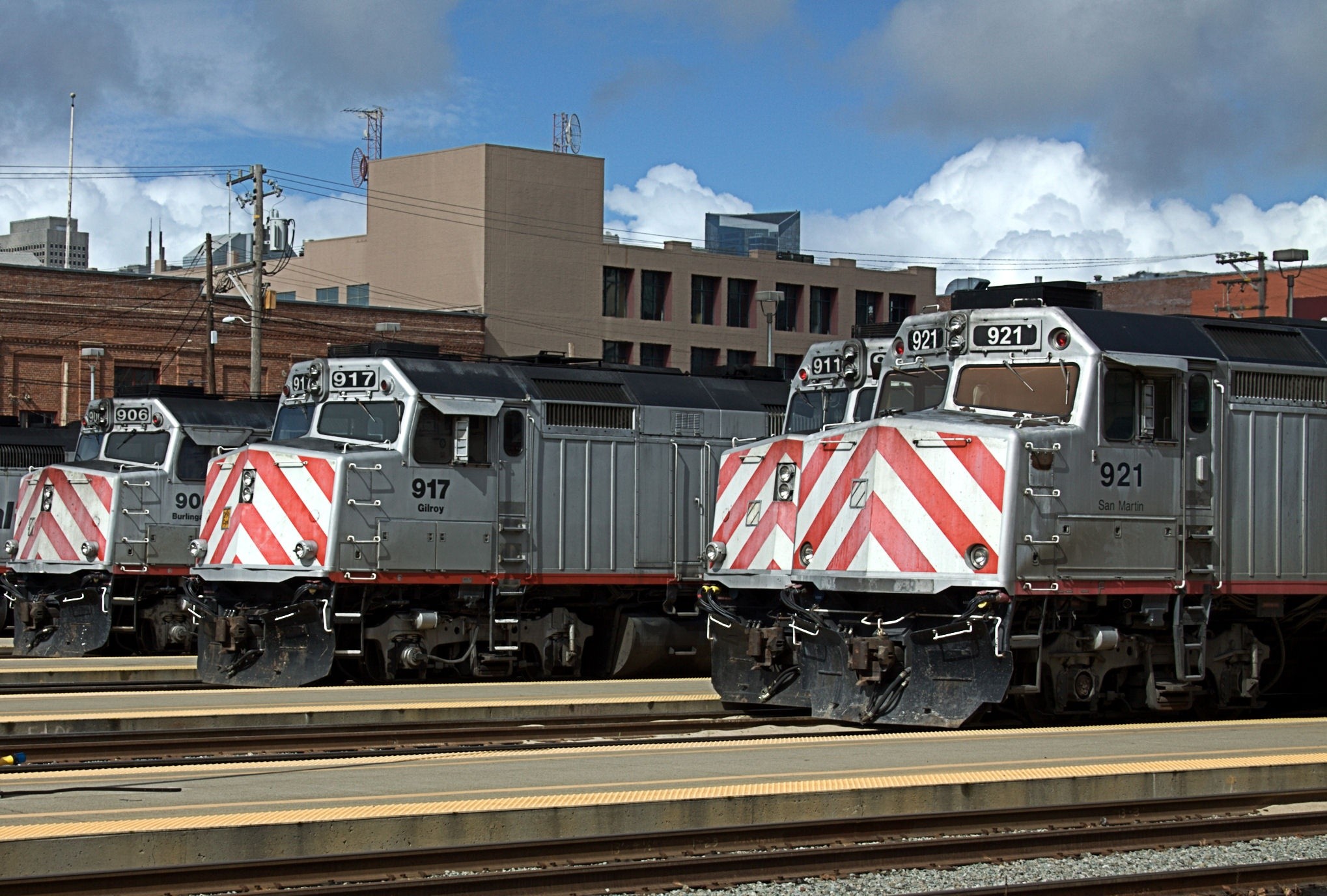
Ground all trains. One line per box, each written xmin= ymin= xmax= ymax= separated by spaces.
xmin=698 ymin=277 xmax=1325 ymax=728
xmin=1 ymin=348 xmax=815 ymax=686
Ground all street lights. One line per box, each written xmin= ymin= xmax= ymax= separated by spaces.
xmin=1273 ymin=249 xmax=1309 ymax=317
xmin=221 ymin=316 xmax=263 ymax=399
xmin=755 ymin=290 xmax=785 ymax=367
xmin=80 ymin=346 xmax=104 ymax=401
